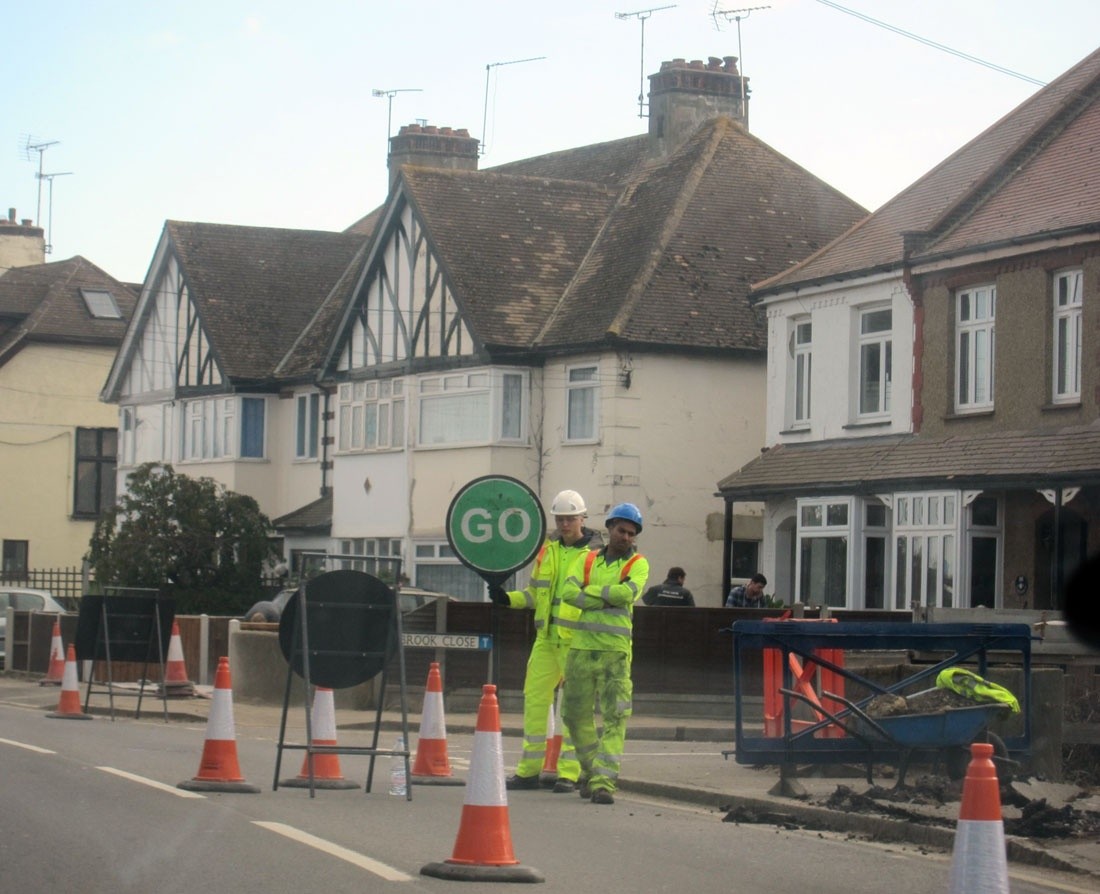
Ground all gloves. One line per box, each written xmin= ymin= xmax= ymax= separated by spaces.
xmin=488 ymin=585 xmax=510 ymax=606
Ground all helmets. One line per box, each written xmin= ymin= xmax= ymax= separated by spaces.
xmin=605 ymin=503 xmax=642 ymax=534
xmin=550 ymin=490 xmax=587 ymax=516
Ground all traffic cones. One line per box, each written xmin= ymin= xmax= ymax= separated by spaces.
xmin=419 ymin=682 xmax=546 ymax=881
xmin=280 ymin=684 xmax=362 ymax=790
xmin=158 ymin=619 xmax=195 ymax=698
xmin=536 ymin=680 xmax=567 ymax=782
xmin=944 ymin=743 xmax=1011 ymax=894
xmin=408 ymin=661 xmax=466 ymax=785
xmin=45 ymin=643 xmax=94 ymax=721
xmin=178 ymin=656 xmax=261 ymax=794
xmin=40 ymin=620 xmax=69 ymax=684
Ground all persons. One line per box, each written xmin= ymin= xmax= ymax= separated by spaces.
xmin=487 ymin=489 xmax=606 ymax=793
xmin=642 ymin=567 xmax=695 ymax=607
xmin=725 ymin=574 xmax=769 ymax=609
xmin=560 ymin=503 xmax=649 ymax=804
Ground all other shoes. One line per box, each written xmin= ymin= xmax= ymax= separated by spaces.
xmin=580 ymin=773 xmax=592 ymax=798
xmin=506 ymin=775 xmax=540 ymax=789
xmin=553 ymin=777 xmax=575 ymax=793
xmin=592 ymin=787 xmax=615 ymax=804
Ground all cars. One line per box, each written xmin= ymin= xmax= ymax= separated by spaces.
xmin=0 ymin=586 xmax=69 ymax=632
